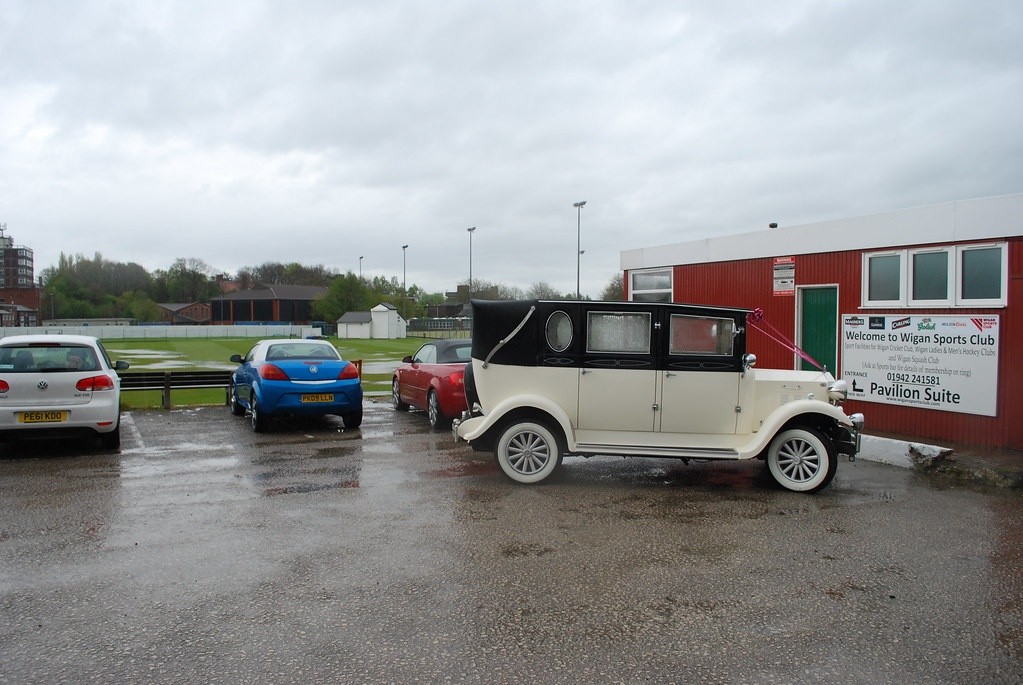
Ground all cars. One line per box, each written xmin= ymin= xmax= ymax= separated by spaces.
xmin=391 ymin=338 xmax=475 ymax=431
xmin=0 ymin=334 xmax=130 ymax=451
xmin=228 ymin=338 xmax=364 ymax=434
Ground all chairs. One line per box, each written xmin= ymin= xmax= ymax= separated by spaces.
xmin=309 ymin=349 xmax=327 ymax=356
xmin=16 ymin=351 xmax=34 ymax=368
xmin=67 ymin=349 xmax=89 ymax=367
xmin=271 ymin=349 xmax=292 ymax=357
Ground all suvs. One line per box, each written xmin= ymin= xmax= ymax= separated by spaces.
xmin=448 ymin=298 xmax=865 ymax=493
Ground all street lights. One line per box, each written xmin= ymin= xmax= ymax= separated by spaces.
xmin=467 ymin=226 xmax=476 ymax=298
xmin=359 ymin=257 xmax=363 ymax=309
xmin=572 ymin=199 xmax=587 ymax=297
xmin=402 ymin=244 xmax=409 ymax=317
